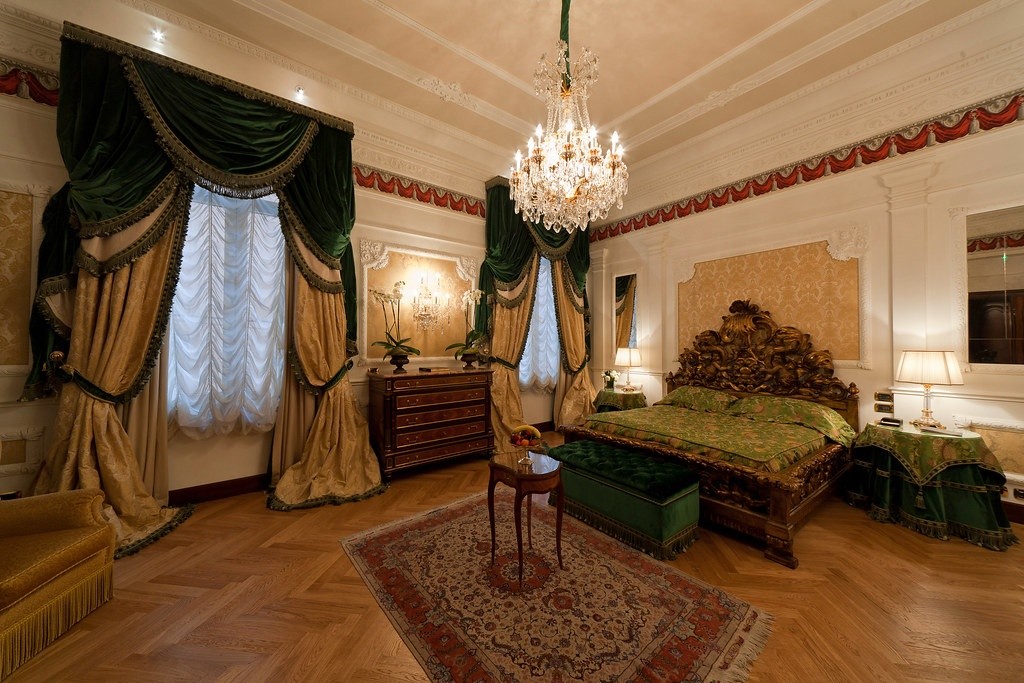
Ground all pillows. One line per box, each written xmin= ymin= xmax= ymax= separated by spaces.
xmin=727 ymin=396 xmax=855 ymax=447
xmin=653 ymin=385 xmax=739 ymax=414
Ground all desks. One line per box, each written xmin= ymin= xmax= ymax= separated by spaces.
xmin=593 ymin=386 xmax=646 ymax=412
xmin=849 ymin=421 xmax=985 ymax=551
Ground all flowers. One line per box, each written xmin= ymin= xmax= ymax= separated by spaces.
xmin=445 ymin=289 xmax=492 ymax=360
xmin=601 ymin=370 xmax=620 ymax=384
xmin=368 ymin=282 xmax=420 ymax=361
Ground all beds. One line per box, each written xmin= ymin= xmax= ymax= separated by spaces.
xmin=558 ymin=299 xmax=859 ymax=570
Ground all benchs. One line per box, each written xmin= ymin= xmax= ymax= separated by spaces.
xmin=547 ymin=439 xmax=699 ymax=560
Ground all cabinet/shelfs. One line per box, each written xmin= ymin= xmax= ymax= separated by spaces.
xmin=368 ymin=368 xmax=497 ymax=488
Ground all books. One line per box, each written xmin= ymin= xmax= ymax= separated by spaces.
xmin=920 ymin=426 xmax=962 ymax=437
xmin=419 ymin=367 xmax=449 ymax=372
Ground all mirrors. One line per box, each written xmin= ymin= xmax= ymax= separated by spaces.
xmin=948 ymin=196 xmax=1024 ymax=374
xmin=611 ymin=267 xmax=641 ymax=360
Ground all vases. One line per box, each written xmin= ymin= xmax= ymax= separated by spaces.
xmin=461 ymin=354 xmax=479 ymax=370
xmin=391 ymin=355 xmax=409 ymax=373
xmin=605 ymin=381 xmax=614 ymax=391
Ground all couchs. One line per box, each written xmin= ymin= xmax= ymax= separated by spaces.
xmin=0 ymin=488 xmax=114 ymax=682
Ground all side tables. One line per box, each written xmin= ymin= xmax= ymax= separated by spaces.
xmin=488 ymin=450 xmax=564 ymax=583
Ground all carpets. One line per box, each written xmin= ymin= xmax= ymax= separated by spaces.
xmin=340 ymin=488 xmax=776 ymax=683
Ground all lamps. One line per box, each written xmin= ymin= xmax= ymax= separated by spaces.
xmin=509 ymin=0 xmax=629 ymax=234
xmin=411 ymin=266 xmax=451 ymax=336
xmin=895 ymin=351 xmax=963 ymax=429
xmin=614 ymin=348 xmax=641 ymax=391
